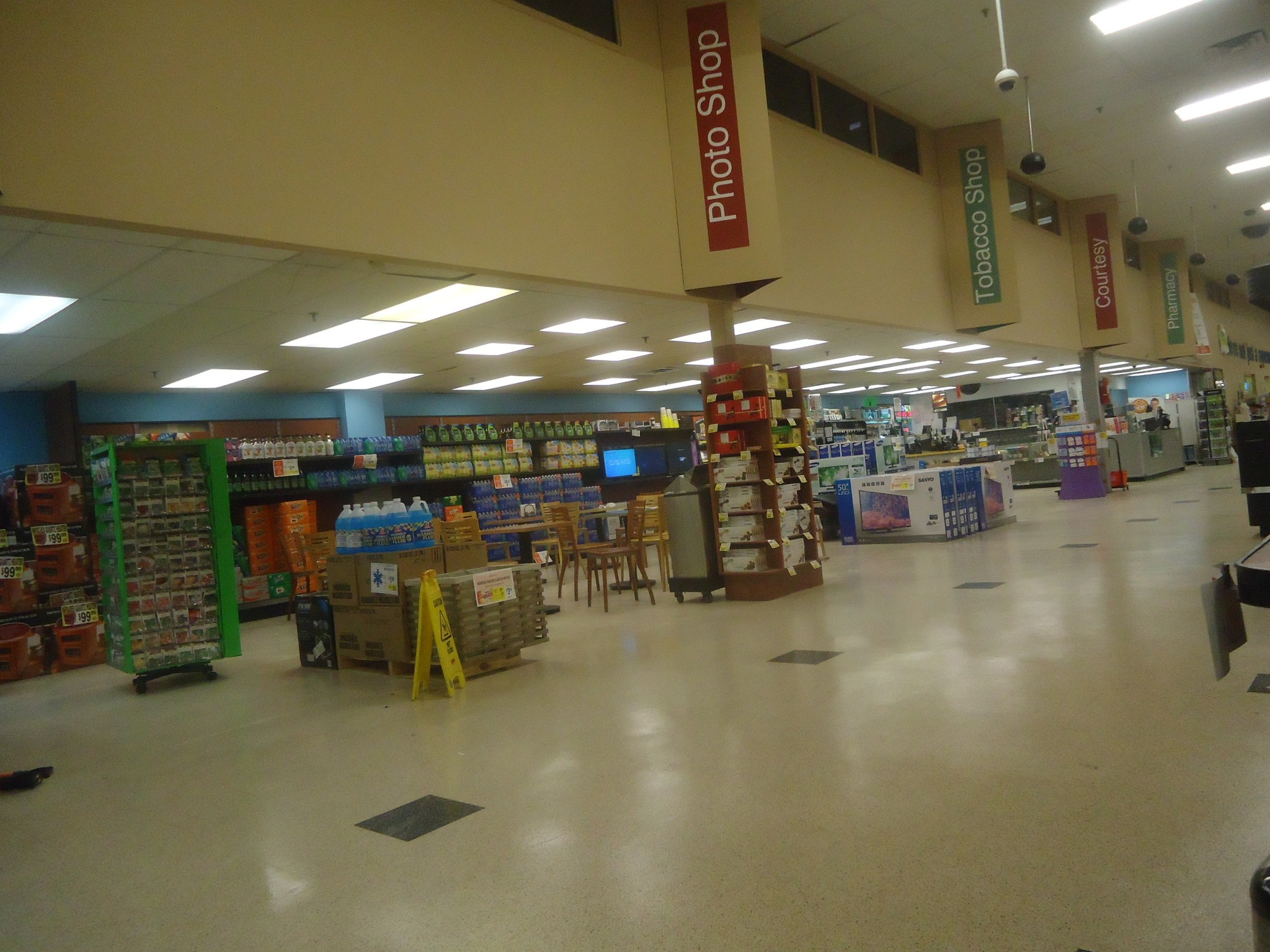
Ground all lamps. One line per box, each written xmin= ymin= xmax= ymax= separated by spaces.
xmin=1020 ymin=76 xmax=1241 ymax=286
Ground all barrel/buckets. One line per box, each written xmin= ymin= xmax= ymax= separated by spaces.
xmin=407 ymin=496 xmax=435 ymax=547
xmin=335 ymin=504 xmax=352 ymax=554
xmin=386 ymin=498 xmax=408 ymax=550
xmin=360 ymin=500 xmax=394 ymax=552
xmin=345 ymin=504 xmax=365 ymax=554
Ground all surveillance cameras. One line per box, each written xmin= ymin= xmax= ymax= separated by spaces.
xmin=994 ymin=69 xmax=1019 ymax=91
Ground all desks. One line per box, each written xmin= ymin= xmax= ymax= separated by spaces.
xmin=480 ymin=522 xmax=572 ymax=615
xmin=584 ymin=507 xmax=659 ymax=590
xmin=483 ymin=516 xmax=553 ymax=584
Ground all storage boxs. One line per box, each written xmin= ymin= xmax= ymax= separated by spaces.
xmin=295 ymin=590 xmax=339 ymax=670
xmin=325 ymin=555 xmax=359 ymax=608
xmin=443 ymin=541 xmax=488 ymax=574
xmin=354 ymin=546 xmax=445 ymax=606
xmin=0 ymin=472 xmax=102 ymax=684
xmin=332 ymin=607 xmax=411 ymax=662
xmin=405 ymin=563 xmax=550 ymax=660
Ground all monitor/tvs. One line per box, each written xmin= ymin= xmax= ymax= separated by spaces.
xmin=601 ymin=447 xmax=638 ymax=479
xmin=984 ymin=478 xmax=1005 ymax=518
xmin=883 ymin=445 xmax=899 ymax=468
xmin=859 ymin=490 xmax=911 ymax=533
xmin=818 ymin=464 xmax=850 ymax=490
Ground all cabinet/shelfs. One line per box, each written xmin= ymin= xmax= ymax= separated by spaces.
xmin=226 ymin=450 xmax=426 ymax=500
xmin=700 ymin=344 xmax=823 ymax=601
xmin=423 ymin=434 xmax=600 ymax=484
xmin=1185 ymin=389 xmax=1232 ymax=462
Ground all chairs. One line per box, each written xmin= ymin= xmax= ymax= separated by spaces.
xmin=433 ymin=491 xmax=670 ymax=620
xmin=278 ymin=530 xmax=336 ymax=620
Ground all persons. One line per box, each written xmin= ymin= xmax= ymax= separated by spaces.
xmin=1150 ymin=398 xmax=1166 ymax=419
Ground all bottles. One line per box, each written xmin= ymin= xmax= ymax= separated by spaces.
xmin=660 ymin=407 xmax=669 ymax=428
xmin=1005 ymin=404 xmax=1046 ymax=428
xmin=418 ymin=419 xmax=601 ymax=479
xmin=464 ymin=473 xmax=606 ymax=562
xmin=672 ymin=412 xmax=679 ymax=428
xmin=666 ymin=409 xmax=674 ymax=428
xmin=681 ymin=416 xmax=688 ymax=428
xmin=221 ymin=434 xmax=423 ymax=492
xmin=832 ymin=427 xmax=867 ymax=445
xmin=678 ymin=418 xmax=684 ymax=428
